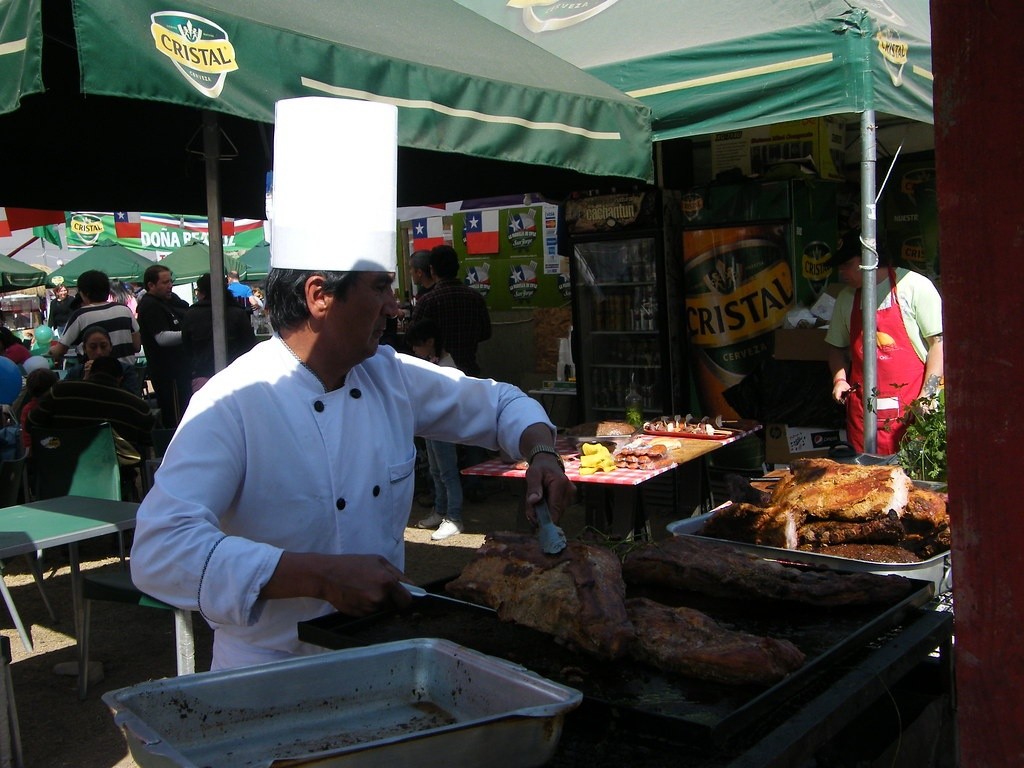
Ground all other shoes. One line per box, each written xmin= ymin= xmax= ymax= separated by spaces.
xmin=418 ymin=493 xmax=436 ymax=507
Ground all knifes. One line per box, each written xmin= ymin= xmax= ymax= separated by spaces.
xmin=398 ymin=580 xmax=499 ymax=613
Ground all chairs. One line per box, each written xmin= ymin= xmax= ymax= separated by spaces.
xmin=27 ymin=419 xmax=211 ymax=704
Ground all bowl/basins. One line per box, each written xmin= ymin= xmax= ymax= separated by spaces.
xmin=576 ymin=441 xmax=617 ymax=456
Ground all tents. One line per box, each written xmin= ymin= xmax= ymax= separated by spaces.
xmin=456 ymin=0 xmax=937 ymax=461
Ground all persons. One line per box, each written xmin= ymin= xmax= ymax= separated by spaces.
xmin=138 ymin=264 xmax=268 ymax=448
xmin=64 ymin=325 xmax=141 ymax=399
xmin=127 ymin=95 xmax=578 ymax=675
xmin=47 ymin=269 xmax=141 ymax=395
xmin=19 ymin=368 xmax=59 ymax=449
xmin=821 ymin=227 xmax=945 ymax=459
xmin=24 ymin=356 xmax=155 ymax=503
xmin=0 ymin=309 xmax=34 ymax=357
xmin=47 ymin=284 xmax=81 ymax=337
xmin=377 ymin=241 xmax=493 ymax=533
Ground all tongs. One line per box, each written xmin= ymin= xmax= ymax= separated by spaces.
xmin=840 ymin=382 xmax=862 ymax=405
xmin=535 ymin=495 xmax=566 ymax=554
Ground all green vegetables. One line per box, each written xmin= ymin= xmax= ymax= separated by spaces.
xmin=865 ymin=381 xmax=947 ymax=483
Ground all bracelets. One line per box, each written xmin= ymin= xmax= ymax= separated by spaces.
xmin=834 ymin=378 xmax=846 ymax=385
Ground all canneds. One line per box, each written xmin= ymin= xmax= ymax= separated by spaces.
xmin=590 ymin=242 xmax=656 ymax=410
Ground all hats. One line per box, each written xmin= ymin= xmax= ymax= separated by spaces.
xmin=825 ymin=230 xmax=879 ymax=266
xmin=271 ymin=97 xmax=399 ymax=273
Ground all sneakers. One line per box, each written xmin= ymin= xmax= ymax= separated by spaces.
xmin=418 ymin=513 xmax=445 ymax=528
xmin=432 ymin=518 xmax=463 ymax=540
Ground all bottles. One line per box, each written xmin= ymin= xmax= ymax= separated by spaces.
xmin=235 ymin=296 xmax=249 ymax=308
xmin=609 ymin=337 xmax=661 ymax=365
xmin=600 ymin=374 xmax=624 ymax=406
xmin=641 ymin=383 xmax=655 ymax=408
xmin=630 ymin=302 xmax=654 ymax=331
xmin=626 ymin=382 xmax=642 ymax=429
xmin=256 ymin=319 xmax=273 ymax=334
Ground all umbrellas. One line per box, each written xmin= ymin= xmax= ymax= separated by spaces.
xmin=0 ymin=0 xmax=654 ymax=378
xmin=0 ymin=253 xmax=47 ymax=294
xmin=237 ymin=238 xmax=275 ymax=282
xmin=159 ymin=238 xmax=248 ymax=305
xmin=43 ymin=238 xmax=156 ymax=290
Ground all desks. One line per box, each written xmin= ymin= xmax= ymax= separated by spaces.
xmin=0 ymin=493 xmax=145 ymax=675
xmin=461 ymin=420 xmax=763 ymax=543
xmin=528 ymin=390 xmax=577 ymax=429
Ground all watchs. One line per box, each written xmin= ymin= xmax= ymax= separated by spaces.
xmin=528 ymin=445 xmax=565 ymax=473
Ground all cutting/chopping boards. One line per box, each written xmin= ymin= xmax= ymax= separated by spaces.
xmin=751 ymin=468 xmax=791 ymax=494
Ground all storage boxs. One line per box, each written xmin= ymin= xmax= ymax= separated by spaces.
xmin=710 ymin=116 xmax=848 ymax=181
xmin=765 ymin=423 xmax=839 ymax=465
xmin=774 ymin=327 xmax=848 ymax=362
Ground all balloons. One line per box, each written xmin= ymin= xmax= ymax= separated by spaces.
xmin=0 ymin=356 xmax=24 ymax=408
xmin=31 ymin=324 xmax=54 ymax=350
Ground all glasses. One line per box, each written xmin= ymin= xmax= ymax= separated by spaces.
xmin=194 ymin=289 xmax=202 ymax=296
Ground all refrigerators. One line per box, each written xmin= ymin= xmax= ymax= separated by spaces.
xmin=565 ymin=185 xmax=690 ymax=424
